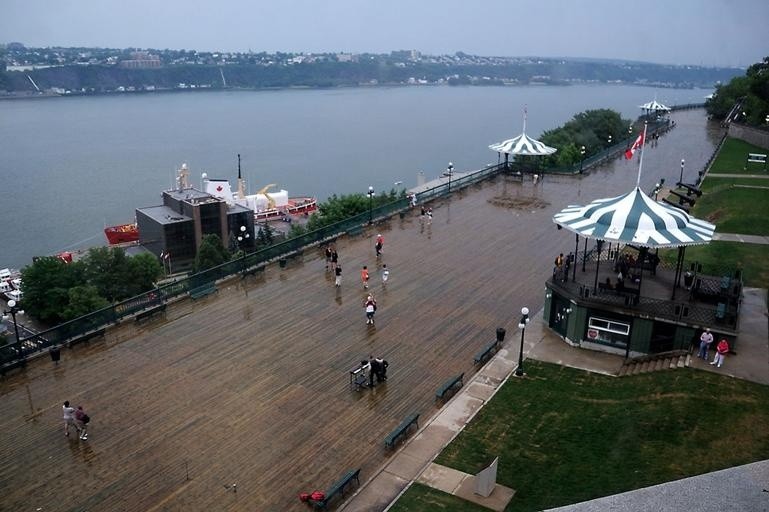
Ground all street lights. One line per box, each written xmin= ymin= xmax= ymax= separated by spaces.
xmin=607 ymin=110 xmax=672 ymax=158
xmin=654 ymin=183 xmax=661 ymax=202
xmin=0 ymin=298 xmax=30 ymax=370
xmin=679 ymin=158 xmax=685 ymax=190
xmin=445 ymin=162 xmax=455 ymax=195
xmin=236 ymin=222 xmax=250 ymax=278
xmin=514 ymin=307 xmax=529 ymax=378
xmin=579 ymin=146 xmax=587 ymax=176
xmin=366 ymin=186 xmax=374 ymax=228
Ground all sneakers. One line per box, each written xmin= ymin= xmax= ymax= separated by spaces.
xmin=717 ymin=363 xmax=721 ymax=368
xmin=367 ymin=319 xmax=370 ymax=324
xmin=371 ymin=319 xmax=374 ymax=324
xmin=80 ymin=437 xmax=87 ymax=440
xmin=710 ymin=362 xmax=717 ymax=365
xmin=84 ymin=434 xmax=88 ymax=437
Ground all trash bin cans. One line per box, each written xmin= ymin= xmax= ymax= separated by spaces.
xmin=279 ymin=258 xmax=286 ymax=268
xmin=496 ymin=328 xmax=506 ymax=346
xmin=49 ymin=347 xmax=60 ymax=365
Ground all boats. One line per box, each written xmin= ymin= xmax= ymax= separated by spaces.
xmin=103 ymin=152 xmax=319 ymax=245
xmin=0 ymin=267 xmax=26 ymax=308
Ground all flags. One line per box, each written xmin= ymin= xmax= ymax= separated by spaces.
xmin=625 ymin=129 xmax=643 ymax=160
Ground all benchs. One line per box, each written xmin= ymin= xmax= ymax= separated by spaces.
xmin=615 ymin=257 xmax=657 ymax=278
xmin=596 ymin=281 xmax=639 ymax=301
xmin=471 ymin=337 xmax=502 ymax=366
xmin=344 ymin=223 xmax=367 ymax=240
xmin=307 ymin=465 xmax=362 ymax=511
xmin=383 ymin=412 xmax=421 ymax=451
xmin=433 ymin=370 xmax=469 ymax=402
xmin=188 ymin=280 xmax=220 ymax=303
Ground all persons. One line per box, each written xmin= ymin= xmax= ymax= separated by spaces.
xmin=709 ymin=336 xmax=730 ymax=368
xmin=361 ymin=266 xmax=370 ymax=289
xmin=62 ymin=400 xmax=80 ymax=436
xmin=366 ymin=354 xmax=384 ymax=388
xmin=697 ymin=327 xmax=714 ymax=361
xmin=365 ymin=293 xmax=377 ymax=322
xmin=375 ymin=234 xmax=383 ymax=258
xmin=326 ymin=244 xmax=343 ymax=289
xmin=407 ymin=191 xmax=433 ymax=226
xmin=382 ymin=264 xmax=389 ymax=285
xmin=363 ymin=295 xmax=376 ymax=325
xmin=377 ymin=356 xmax=390 ymax=382
xmin=564 ymin=255 xmax=571 ymax=282
xmin=683 ymin=269 xmax=691 ymax=291
xmin=603 ymin=247 xmax=641 ymax=298
xmin=555 ymin=252 xmax=563 ymax=280
xmin=74 ymin=404 xmax=90 ymax=441
